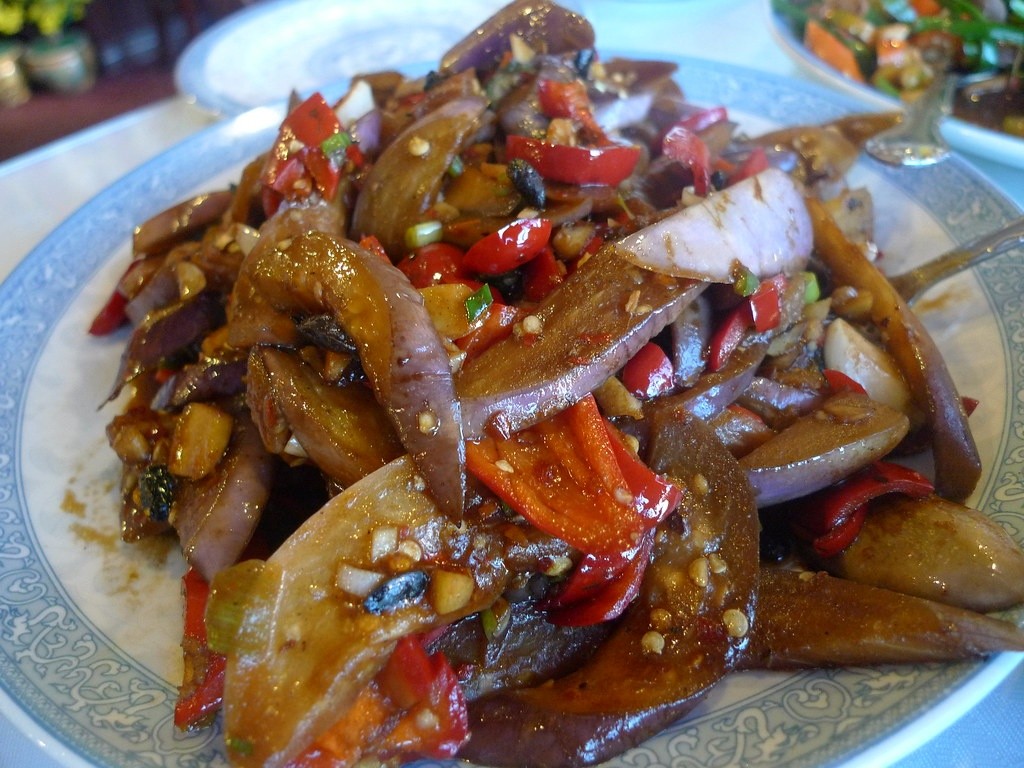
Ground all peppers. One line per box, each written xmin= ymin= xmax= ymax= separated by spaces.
xmin=173 ymin=92 xmax=932 ymax=734
xmin=773 ymin=0 xmax=1024 ymax=98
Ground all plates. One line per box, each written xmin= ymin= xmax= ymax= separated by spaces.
xmin=0 ymin=63 xmax=1024 ymax=768
xmin=761 ymin=0 xmax=1024 ymax=168
xmin=173 ymin=1 xmax=781 ymax=114
